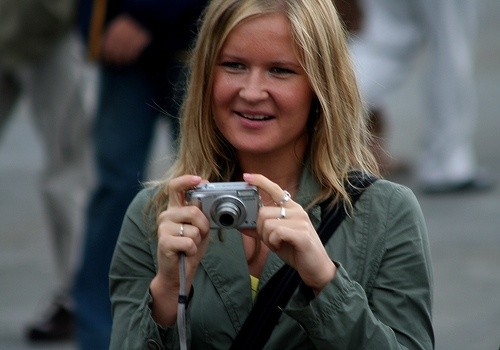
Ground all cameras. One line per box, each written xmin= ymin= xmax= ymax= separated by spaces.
xmin=186 ymin=181 xmax=259 ymax=229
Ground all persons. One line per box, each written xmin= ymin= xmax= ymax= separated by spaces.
xmin=106 ymin=0 xmax=435 ymax=350
xmin=74 ymin=0 xmax=210 ymax=350
xmin=343 ymin=0 xmax=500 ymax=195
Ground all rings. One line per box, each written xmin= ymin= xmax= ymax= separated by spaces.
xmin=178 ymin=224 xmax=184 ymax=236
xmin=274 ymin=191 xmax=291 ymax=206
xmin=281 ymin=206 xmax=285 ymax=219
